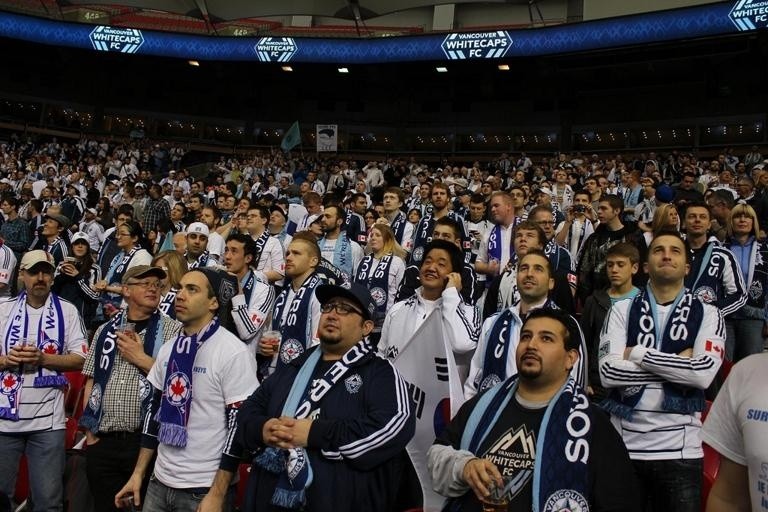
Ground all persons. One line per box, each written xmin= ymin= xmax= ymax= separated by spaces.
xmin=0 ymin=133 xmax=768 ymax=512
